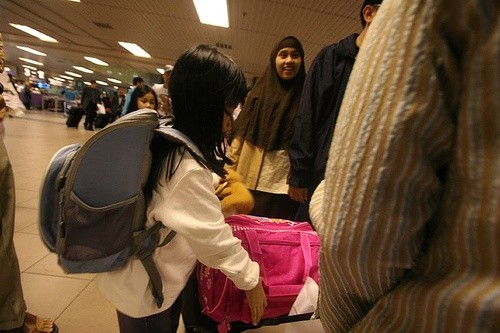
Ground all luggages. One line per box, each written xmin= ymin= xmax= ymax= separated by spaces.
xmin=66 ymin=105 xmax=82 ymax=129
xmin=95 ymin=111 xmax=109 ymax=130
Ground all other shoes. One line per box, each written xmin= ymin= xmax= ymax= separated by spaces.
xmin=84 ymin=122 xmax=89 ymax=130
xmin=90 ymin=126 xmax=94 ymax=131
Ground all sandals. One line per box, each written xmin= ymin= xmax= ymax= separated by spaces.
xmin=11 ymin=312 xmax=58 ymax=333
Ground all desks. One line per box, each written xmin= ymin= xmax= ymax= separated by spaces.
xmin=42 ymin=95 xmax=65 ymax=113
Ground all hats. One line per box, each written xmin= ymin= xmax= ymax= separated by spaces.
xmin=165 ymin=64 xmax=173 ymax=72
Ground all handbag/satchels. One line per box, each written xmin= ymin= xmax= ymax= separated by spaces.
xmin=96 ymin=102 xmax=106 ymax=114
xmin=196 ymin=214 xmax=321 ymax=333
xmin=85 ymin=101 xmax=95 ymax=113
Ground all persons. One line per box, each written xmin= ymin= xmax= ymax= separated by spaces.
xmin=95 ymin=44 xmax=268 ymax=333
xmin=309 ymin=0 xmax=500 ymax=333
xmin=0 ymin=33 xmax=59 ymax=333
xmin=224 ymin=36 xmax=306 ymax=219
xmin=1 ymin=73 xmax=253 ymax=159
xmin=288 ymin=0 xmax=384 ymax=224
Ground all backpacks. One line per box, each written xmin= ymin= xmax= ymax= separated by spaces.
xmin=37 ymin=108 xmax=208 ymax=274
